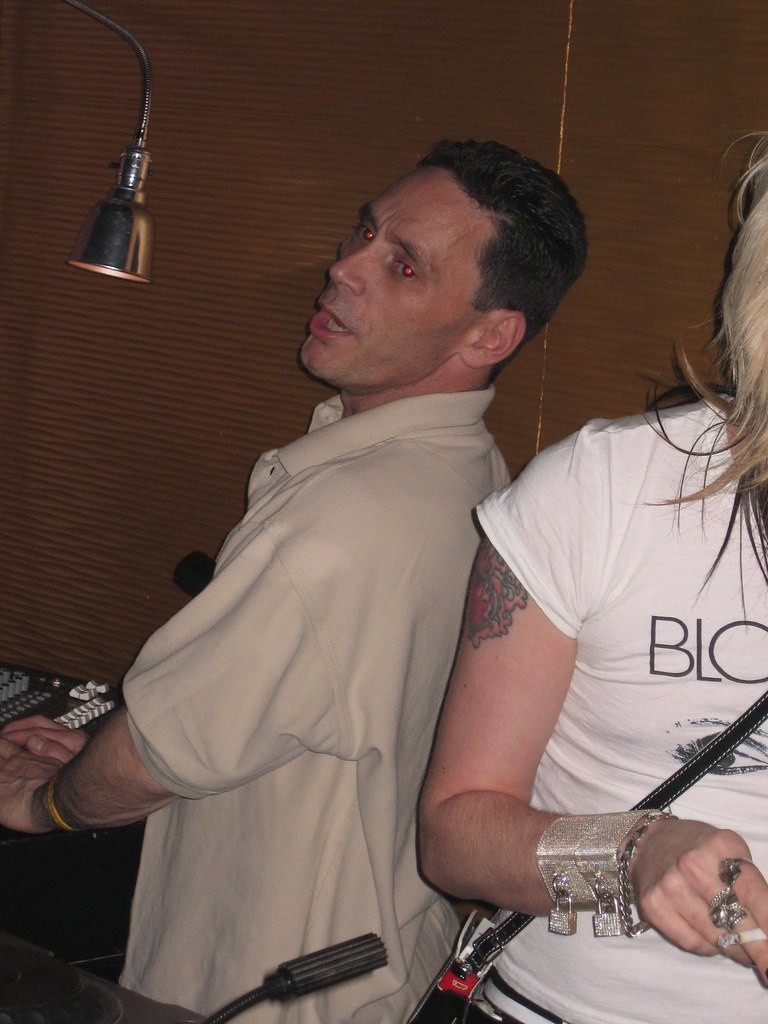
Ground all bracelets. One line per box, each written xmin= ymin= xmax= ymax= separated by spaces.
xmin=48 ymin=774 xmax=83 ymax=832
xmin=536 ymin=809 xmax=679 ymax=938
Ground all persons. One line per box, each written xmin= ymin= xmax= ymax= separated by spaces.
xmin=0 ymin=139 xmax=588 ymax=1024
xmin=417 ymin=148 xmax=768 ymax=1024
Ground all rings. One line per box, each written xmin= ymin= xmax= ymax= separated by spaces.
xmin=717 ymin=857 xmax=755 ymax=887
xmin=710 ymin=888 xmax=749 ymax=932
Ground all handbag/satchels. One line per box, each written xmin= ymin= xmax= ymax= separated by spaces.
xmin=407 ymin=909 xmax=501 ymax=1024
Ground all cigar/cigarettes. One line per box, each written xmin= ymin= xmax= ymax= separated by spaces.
xmin=718 ymin=929 xmax=767 ymax=947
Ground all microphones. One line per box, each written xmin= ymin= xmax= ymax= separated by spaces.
xmin=204 ymin=932 xmax=388 ymax=1024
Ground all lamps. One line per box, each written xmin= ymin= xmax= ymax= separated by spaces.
xmin=53 ymin=1 xmax=157 ymax=287
